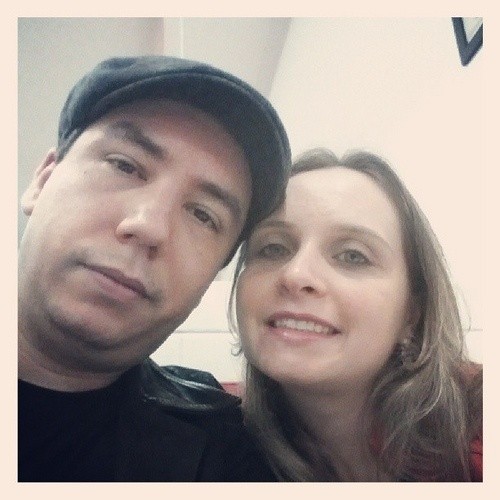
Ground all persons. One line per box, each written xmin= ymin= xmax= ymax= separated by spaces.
xmin=228 ymin=148 xmax=483 ymax=483
xmin=18 ymin=54 xmax=279 ymax=482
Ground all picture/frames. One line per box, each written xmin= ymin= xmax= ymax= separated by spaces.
xmin=451 ymin=16 xmax=483 ymax=66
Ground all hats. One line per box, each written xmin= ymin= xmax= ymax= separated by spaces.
xmin=59 ymin=56 xmax=291 ymax=222
